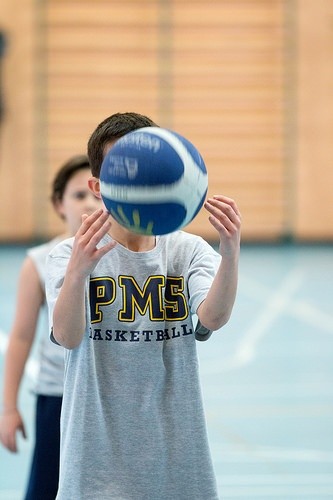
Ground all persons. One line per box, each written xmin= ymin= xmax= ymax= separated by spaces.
xmin=43 ymin=111 xmax=242 ymax=500
xmin=1 ymin=154 xmax=109 ymax=500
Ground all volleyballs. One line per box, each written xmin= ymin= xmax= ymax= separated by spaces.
xmin=98 ymin=125 xmax=210 ymax=236
